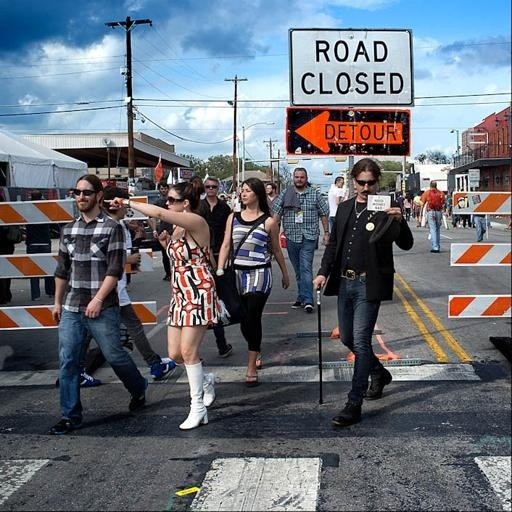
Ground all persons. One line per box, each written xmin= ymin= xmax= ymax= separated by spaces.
xmin=0 ymin=189 xmax=76 ymax=305
xmin=379 ymin=189 xmax=490 ymax=243
xmin=420 ymin=180 xmax=446 ymax=253
xmin=54 ymin=188 xmax=177 ymax=387
xmin=147 ymin=176 xmax=282 ymax=358
xmin=327 ymin=177 xmax=346 ymax=233
xmin=217 ymin=178 xmax=289 ymax=387
xmin=273 ymin=167 xmax=330 ymax=313
xmin=46 ymin=174 xmax=149 ymax=434
xmin=311 ymin=158 xmax=414 ymax=428
xmin=102 ymin=176 xmax=231 ymax=430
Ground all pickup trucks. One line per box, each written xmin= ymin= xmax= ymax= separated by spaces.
xmin=125 ymin=190 xmax=166 ymax=249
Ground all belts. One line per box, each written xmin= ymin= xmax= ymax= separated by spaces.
xmin=338 ymin=271 xmax=367 ymax=280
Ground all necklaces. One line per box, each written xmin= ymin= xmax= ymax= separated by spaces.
xmin=353 ymin=197 xmax=368 ymax=220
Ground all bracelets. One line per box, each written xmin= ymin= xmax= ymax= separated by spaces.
xmin=122 ymin=199 xmax=132 ymax=209
xmin=325 ymin=229 xmax=329 ymax=233
xmin=96 ymin=298 xmax=105 ymax=305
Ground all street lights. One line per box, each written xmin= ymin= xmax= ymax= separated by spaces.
xmin=450 ymin=128 xmax=461 ymax=155
xmin=126 ymin=114 xmax=145 ymax=179
xmin=240 ymin=121 xmax=275 ymax=182
xmin=227 ymin=100 xmax=237 ymax=195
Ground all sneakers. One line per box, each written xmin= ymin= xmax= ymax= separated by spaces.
xmin=290 ymin=301 xmax=305 ymax=310
xmin=78 ymin=368 xmax=101 ymax=388
xmin=49 ymin=414 xmax=84 ymax=435
xmin=304 ymin=303 xmax=314 ymax=313
xmin=219 ymin=344 xmax=233 ymax=357
xmin=150 ymin=354 xmax=179 ymax=384
xmin=430 ymin=249 xmax=440 ymax=253
xmin=129 ymin=379 xmax=149 ymax=413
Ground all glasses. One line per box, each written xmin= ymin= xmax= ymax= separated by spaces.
xmin=354 ymin=176 xmax=376 ymax=186
xmin=167 ymin=196 xmax=185 ymax=205
xmin=73 ymin=189 xmax=99 ymax=196
xmin=205 ymin=184 xmax=218 ymax=189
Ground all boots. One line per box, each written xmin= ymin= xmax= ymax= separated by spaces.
xmin=175 ymin=361 xmax=217 ymax=407
xmin=178 ymin=361 xmax=209 ymax=431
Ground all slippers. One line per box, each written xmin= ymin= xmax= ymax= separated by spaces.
xmin=244 ymin=370 xmax=258 ymax=387
xmin=255 ymin=353 xmax=262 ymax=369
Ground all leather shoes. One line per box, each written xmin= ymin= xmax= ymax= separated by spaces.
xmin=331 ymin=405 xmax=362 ymax=426
xmin=364 ymin=368 xmax=392 ymax=400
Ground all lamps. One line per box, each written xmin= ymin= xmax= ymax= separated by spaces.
xmin=495 ymin=115 xmax=501 ymax=127
xmin=502 ymin=109 xmax=511 ymax=122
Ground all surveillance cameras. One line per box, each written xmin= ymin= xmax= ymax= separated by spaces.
xmin=120 ymin=66 xmax=126 ymax=76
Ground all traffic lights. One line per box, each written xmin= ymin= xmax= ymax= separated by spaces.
xmin=402 ymin=182 xmax=406 ymax=191
xmin=324 ymin=172 xmax=333 ymax=176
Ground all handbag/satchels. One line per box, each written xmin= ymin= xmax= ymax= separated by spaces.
xmin=206 ymin=270 xmax=249 ymax=329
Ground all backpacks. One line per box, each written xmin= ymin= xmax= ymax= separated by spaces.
xmin=428 ymin=189 xmax=442 ymax=211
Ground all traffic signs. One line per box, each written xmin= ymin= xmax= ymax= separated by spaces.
xmin=283 ymin=105 xmax=415 ymax=158
xmin=283 ymin=24 xmax=417 ymax=109
